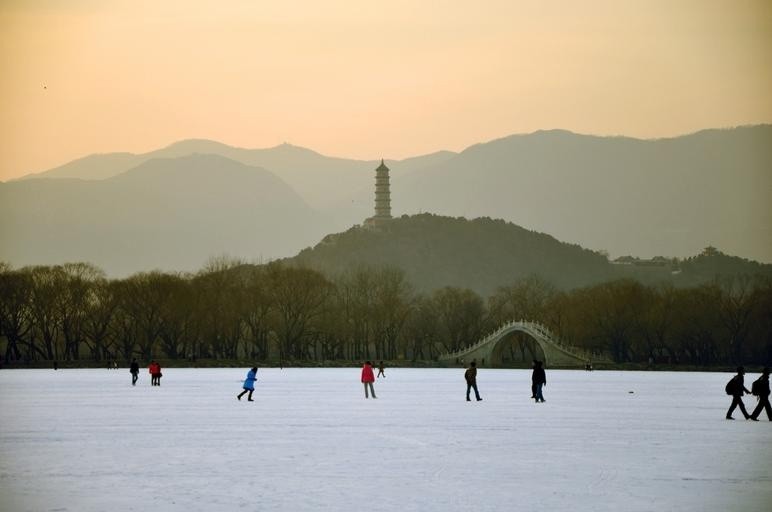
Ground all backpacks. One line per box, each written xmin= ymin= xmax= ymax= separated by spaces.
xmin=726 ymin=378 xmax=736 ymax=394
xmin=752 ymin=381 xmax=761 ymax=396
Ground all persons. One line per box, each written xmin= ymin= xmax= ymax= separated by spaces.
xmin=108 ymin=357 xmax=163 ymax=386
xmin=531 ymin=359 xmax=546 ymax=403
xmin=361 ymin=361 xmax=377 ymax=399
xmin=378 ymin=360 xmax=386 ymax=378
xmin=465 ymin=361 xmax=482 ymax=401
xmin=725 ymin=365 xmax=751 ymax=420
xmin=750 ymin=367 xmax=772 ymax=422
xmin=237 ymin=366 xmax=258 ymax=401
xmin=585 ymin=359 xmax=592 ymax=371
